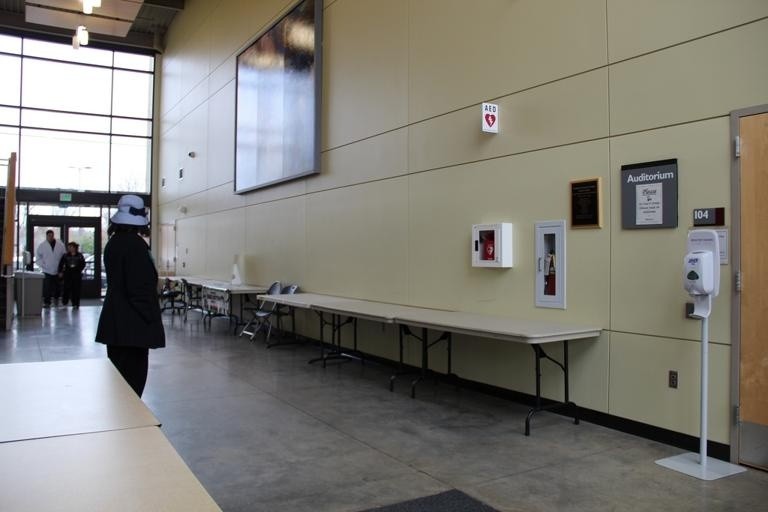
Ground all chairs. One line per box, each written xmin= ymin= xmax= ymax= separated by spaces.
xmin=181 ymin=279 xmax=203 ymax=321
xmin=239 ymin=281 xmax=282 ymax=341
xmin=255 ymin=285 xmax=299 ymax=340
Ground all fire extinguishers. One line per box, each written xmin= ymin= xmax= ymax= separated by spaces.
xmin=544 ymin=248 xmax=557 ymax=299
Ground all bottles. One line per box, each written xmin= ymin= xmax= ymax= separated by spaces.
xmin=232 ymin=264 xmax=241 ymax=285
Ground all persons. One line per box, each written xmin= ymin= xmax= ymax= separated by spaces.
xmin=37 ymin=230 xmax=65 ymax=308
xmin=95 ymin=195 xmax=165 ymax=399
xmin=58 ymin=243 xmax=86 ymax=309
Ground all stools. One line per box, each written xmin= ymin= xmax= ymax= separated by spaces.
xmin=161 ymin=290 xmax=180 ymax=315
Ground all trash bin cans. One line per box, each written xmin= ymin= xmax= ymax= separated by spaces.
xmin=14 ymin=271 xmax=46 ymax=318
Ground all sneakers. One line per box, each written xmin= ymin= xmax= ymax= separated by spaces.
xmin=72 ymin=307 xmax=79 ymax=310
xmin=62 ymin=306 xmax=67 ymax=311
xmin=44 ymin=305 xmax=50 ymax=308
xmin=54 ymin=304 xmax=58 ymax=308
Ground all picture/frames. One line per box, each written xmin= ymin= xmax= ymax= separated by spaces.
xmin=567 ymin=177 xmax=602 ymax=230
xmin=235 ymin=1 xmax=322 ymax=197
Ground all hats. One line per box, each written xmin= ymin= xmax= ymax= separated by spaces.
xmin=110 ymin=195 xmax=149 ymax=226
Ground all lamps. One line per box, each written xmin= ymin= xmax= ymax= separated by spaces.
xmin=72 ymin=27 xmax=89 ymax=48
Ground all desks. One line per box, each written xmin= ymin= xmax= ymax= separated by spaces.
xmin=203 ymin=280 xmax=268 ymax=336
xmin=158 ymin=276 xmax=191 ymax=307
xmin=395 ymin=312 xmax=602 ymax=436
xmin=0 ymin=358 xmax=162 ymax=443
xmin=180 ymin=280 xmax=213 ymax=311
xmin=256 ymin=292 xmax=353 ymax=364
xmin=311 ymin=300 xmax=450 ymax=392
xmin=0 ymin=426 xmax=224 ymax=511
